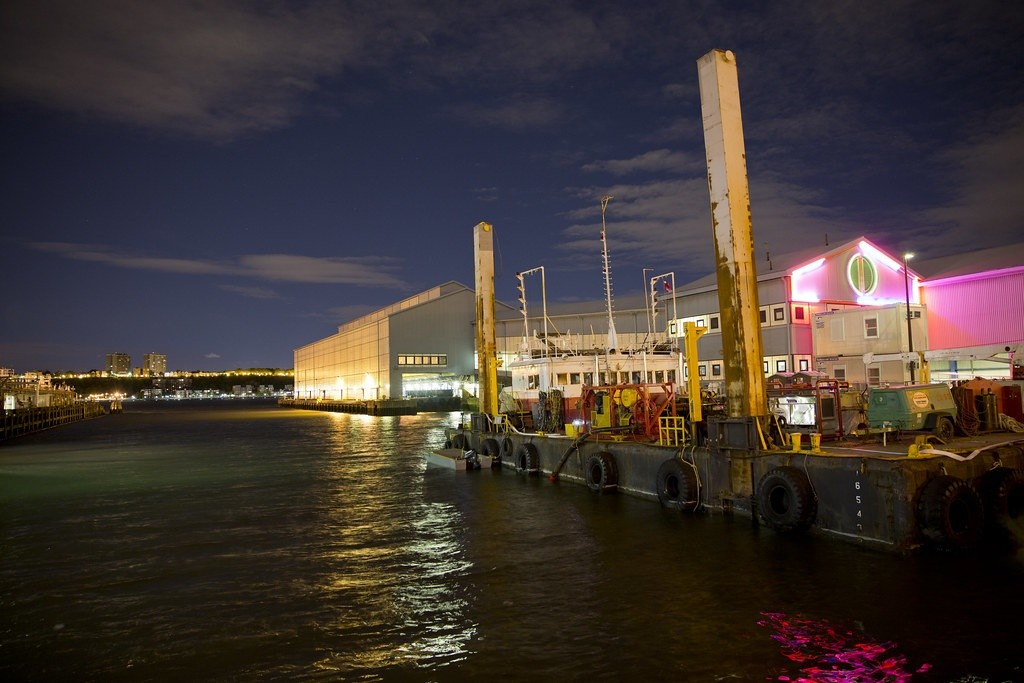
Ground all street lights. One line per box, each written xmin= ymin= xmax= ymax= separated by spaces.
xmin=903 ymin=252 xmax=917 ymax=386
